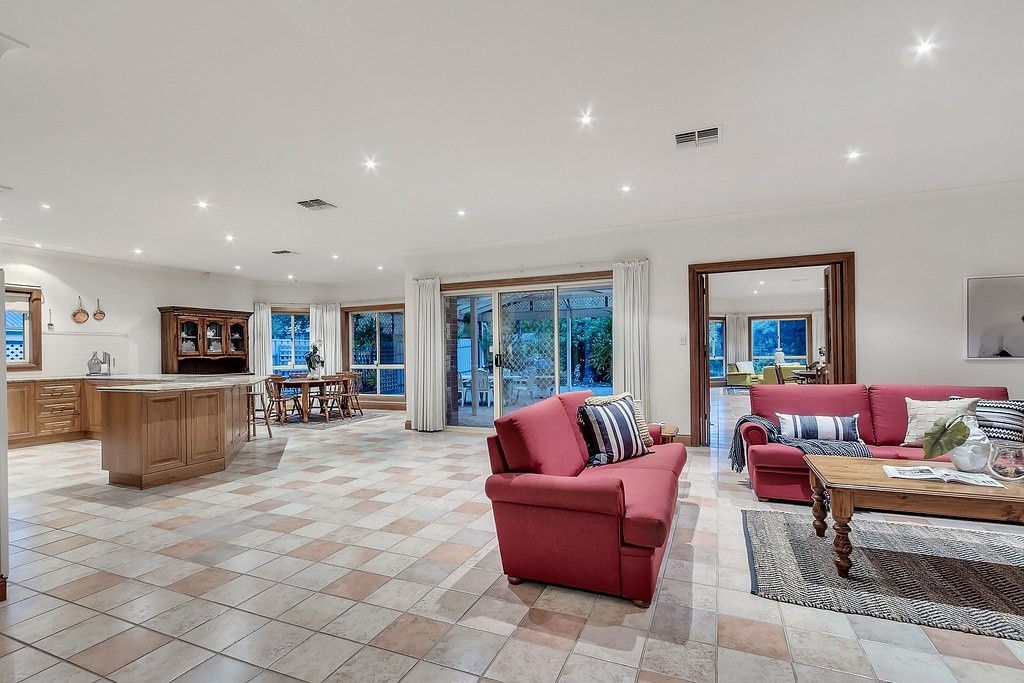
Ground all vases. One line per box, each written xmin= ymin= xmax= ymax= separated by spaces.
xmin=774 ymin=348 xmax=785 ymax=363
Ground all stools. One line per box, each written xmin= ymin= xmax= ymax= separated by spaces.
xmin=245 ymin=392 xmax=272 ymax=441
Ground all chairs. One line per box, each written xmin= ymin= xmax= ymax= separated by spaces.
xmin=773 ymin=361 xmax=827 ymax=383
xmin=266 ymin=371 xmax=363 ymax=423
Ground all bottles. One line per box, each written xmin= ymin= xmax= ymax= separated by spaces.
xmin=87 ymin=351 xmax=103 ymax=374
xmin=986 ymin=440 xmax=1024 ymax=483
xmin=774 ymin=348 xmax=785 ymax=363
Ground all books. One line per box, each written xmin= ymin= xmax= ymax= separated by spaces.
xmin=883 ymin=466 xmax=1007 ymax=488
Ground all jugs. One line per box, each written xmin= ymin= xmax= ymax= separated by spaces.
xmin=206 ymin=326 xmax=216 ymax=338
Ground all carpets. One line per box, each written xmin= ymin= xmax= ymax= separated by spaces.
xmin=742 ymin=509 xmax=1024 ymax=642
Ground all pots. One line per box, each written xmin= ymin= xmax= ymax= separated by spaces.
xmin=70 ymin=295 xmax=89 ymax=323
xmin=92 ymin=299 xmax=105 ymax=320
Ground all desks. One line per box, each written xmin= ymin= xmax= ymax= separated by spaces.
xmin=791 ymin=370 xmax=821 ymax=383
xmin=276 ymin=378 xmax=352 ymax=422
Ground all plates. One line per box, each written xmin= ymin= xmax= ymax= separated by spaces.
xmin=210 ymin=341 xmax=221 ymax=351
xmin=183 ymin=341 xmax=195 ymax=351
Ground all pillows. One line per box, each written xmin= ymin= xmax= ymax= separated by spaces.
xmin=586 ymin=393 xmax=654 ymax=447
xmin=577 ymin=394 xmax=649 ymax=467
xmin=774 ymin=411 xmax=861 ymax=442
xmin=949 ymin=395 xmax=1024 ymax=442
xmin=900 ymin=397 xmax=989 ymax=447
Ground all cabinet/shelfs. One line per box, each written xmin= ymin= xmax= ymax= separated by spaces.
xmin=156 ymin=306 xmax=254 ymax=374
xmin=7 ymin=380 xmax=132 ymax=449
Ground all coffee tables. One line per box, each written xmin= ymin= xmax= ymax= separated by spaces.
xmin=802 ymin=454 xmax=1024 ymax=580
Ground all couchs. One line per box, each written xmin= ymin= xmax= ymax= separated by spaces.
xmin=757 ymin=365 xmax=806 ymax=384
xmin=726 ymin=372 xmax=751 ymax=389
xmin=487 ymin=390 xmax=688 ymax=607
xmin=739 ymin=384 xmax=1008 ymax=501
